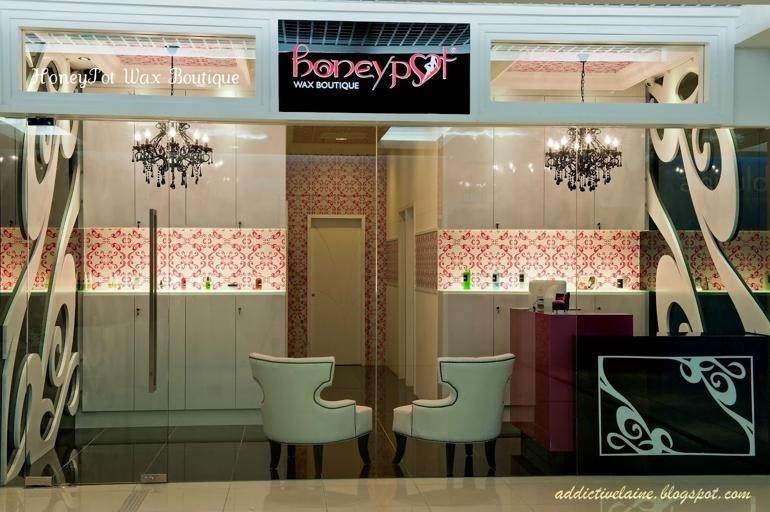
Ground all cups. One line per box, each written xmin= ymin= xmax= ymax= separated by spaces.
xmin=617 ymin=278 xmax=623 ymax=289
xmin=763 ymin=269 xmax=770 ymax=290
xmin=529 ymin=295 xmax=545 ymax=313
xmin=700 ymin=276 xmax=708 ymax=289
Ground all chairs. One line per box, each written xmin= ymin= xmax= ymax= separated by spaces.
xmin=388 ymin=349 xmax=516 ymax=477
xmin=247 ymin=349 xmax=376 ymax=480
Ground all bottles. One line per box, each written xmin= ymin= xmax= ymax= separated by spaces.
xmin=492 ymin=265 xmax=499 ymax=290
xmin=463 ymin=265 xmax=471 ymax=290
xmin=587 ymin=276 xmax=595 ymax=288
xmin=518 ymin=265 xmax=525 ymax=289
xmin=44 ymin=274 xmax=51 ymax=290
xmin=86 ymin=273 xmax=263 ymax=290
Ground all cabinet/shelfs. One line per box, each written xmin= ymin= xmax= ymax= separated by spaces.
xmin=440 ymin=95 xmax=545 ymax=230
xmin=82 ymin=85 xmax=186 ymax=227
xmin=79 ymin=292 xmax=185 ymax=412
xmin=187 ymin=88 xmax=287 ymax=228
xmin=545 ymin=95 xmax=649 ymax=231
xmin=566 ymin=291 xmax=648 ymax=337
xmin=439 ymin=292 xmax=536 ymax=404
xmin=186 ymin=292 xmax=288 ymax=408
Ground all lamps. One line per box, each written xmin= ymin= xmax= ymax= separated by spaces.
xmin=129 ymin=44 xmax=215 ymax=190
xmin=542 ymin=53 xmax=624 ymax=192
